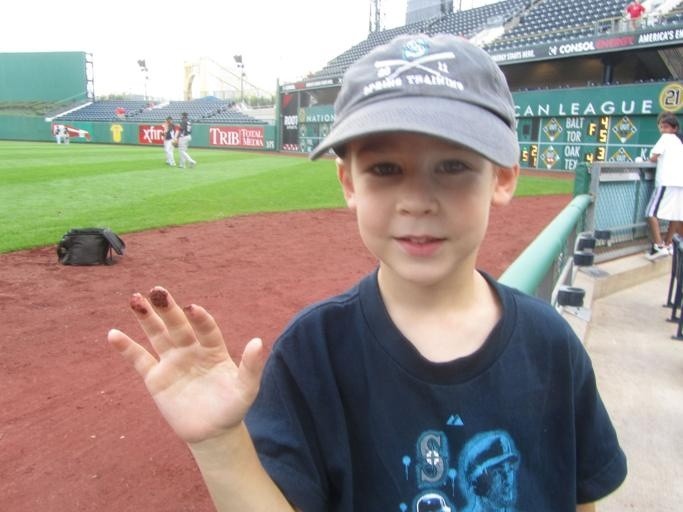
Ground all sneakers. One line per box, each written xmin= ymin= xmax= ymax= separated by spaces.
xmin=644 ymin=241 xmax=673 ymax=260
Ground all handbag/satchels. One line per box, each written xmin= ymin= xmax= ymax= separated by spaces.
xmin=57 ymin=227 xmax=125 ymax=266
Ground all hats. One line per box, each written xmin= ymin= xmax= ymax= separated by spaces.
xmin=310 ymin=34 xmax=520 ymax=169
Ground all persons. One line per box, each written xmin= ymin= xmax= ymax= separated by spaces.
xmin=159 ymin=117 xmax=176 ymax=166
xmin=55 ymin=125 xmax=61 ymax=143
xmin=645 ymin=116 xmax=683 ymax=261
xmin=626 ymin=0 xmax=645 ymax=30
xmin=656 ymin=111 xmax=683 ymax=251
xmin=176 ymin=112 xmax=196 ymax=168
xmin=108 ymin=33 xmax=628 ymax=512
xmin=63 ymin=128 xmax=69 ymax=144
